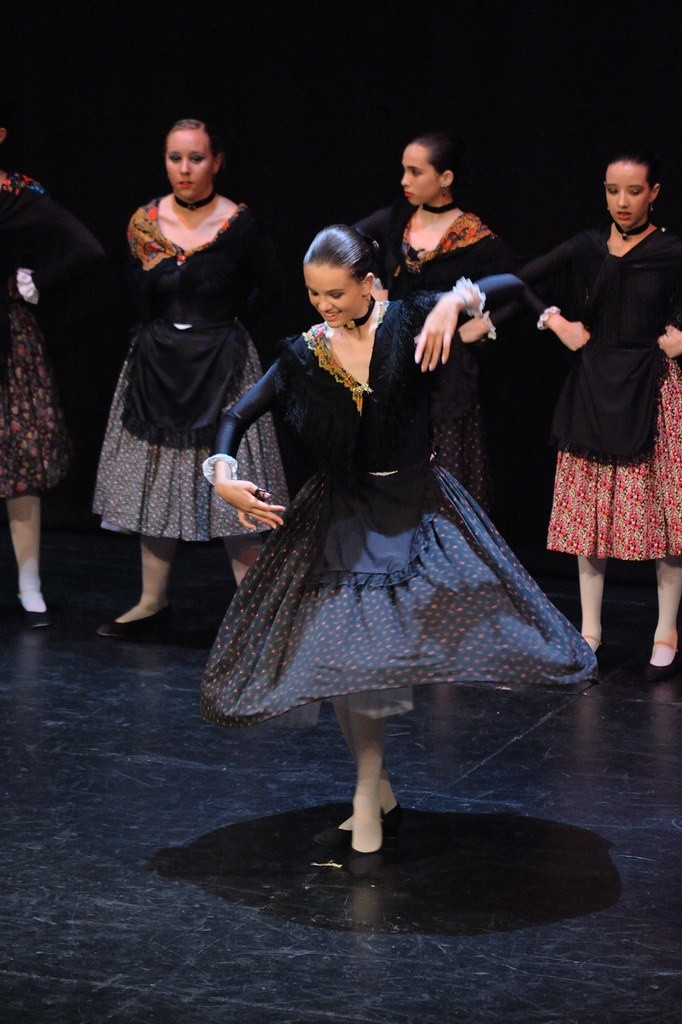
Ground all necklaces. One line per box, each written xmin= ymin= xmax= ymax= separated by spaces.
xmin=423 ymin=201 xmax=460 ymax=214
xmin=343 ymin=294 xmax=375 ymax=329
xmin=175 ymin=189 xmax=216 ymax=210
xmin=615 ymin=217 xmax=650 ymax=240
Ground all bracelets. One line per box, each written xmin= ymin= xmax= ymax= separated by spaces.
xmin=458 ymin=294 xmax=467 ymax=312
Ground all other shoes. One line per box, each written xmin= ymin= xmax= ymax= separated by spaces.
xmin=97 ymin=605 xmax=173 ymax=637
xmin=645 ymin=631 xmax=682 ymax=681
xmin=17 ymin=596 xmax=53 ymax=628
xmin=344 ymin=805 xmax=387 ymax=878
xmin=590 ymin=642 xmax=604 ymax=662
xmin=315 ymin=802 xmax=401 ymax=843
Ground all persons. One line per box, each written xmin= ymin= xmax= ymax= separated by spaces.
xmin=100 ymin=112 xmax=293 ymax=641
xmin=355 ymin=133 xmax=514 ymax=524
xmin=513 ymin=151 xmax=682 ymax=686
xmin=201 ymin=224 xmax=525 ymax=880
xmin=0 ymin=118 xmax=104 ymax=631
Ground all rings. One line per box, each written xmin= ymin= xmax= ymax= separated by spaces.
xmin=254 ymin=488 xmax=267 ymax=502
